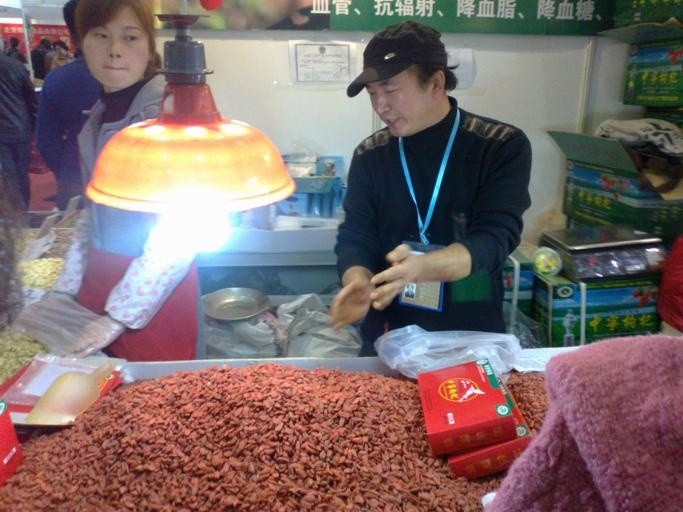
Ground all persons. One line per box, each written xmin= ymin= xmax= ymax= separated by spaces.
xmin=403 ymin=282 xmax=415 ymax=299
xmin=0 ymin=0 xmax=102 ymax=214
xmin=266 ymin=1 xmax=328 ymax=30
xmin=17 ymin=0 xmax=207 ymax=366
xmin=329 ymin=21 xmax=534 ymax=355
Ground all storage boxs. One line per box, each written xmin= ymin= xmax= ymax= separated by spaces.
xmin=533 ymin=128 xmax=679 ymax=351
xmin=597 ymin=16 xmax=683 ymax=110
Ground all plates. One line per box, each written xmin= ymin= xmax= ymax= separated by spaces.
xmin=202 ymin=287 xmax=270 ymax=321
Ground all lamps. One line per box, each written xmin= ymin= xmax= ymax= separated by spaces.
xmin=85 ymin=2 xmax=296 ymax=214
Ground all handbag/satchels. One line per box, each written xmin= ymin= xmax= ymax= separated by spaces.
xmin=621 ymin=140 xmax=683 ymax=179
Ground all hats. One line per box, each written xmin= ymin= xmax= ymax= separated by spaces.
xmin=347 ymin=21 xmax=447 ymax=96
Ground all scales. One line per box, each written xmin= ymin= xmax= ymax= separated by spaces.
xmin=537 ymin=223 xmax=669 ymax=282
